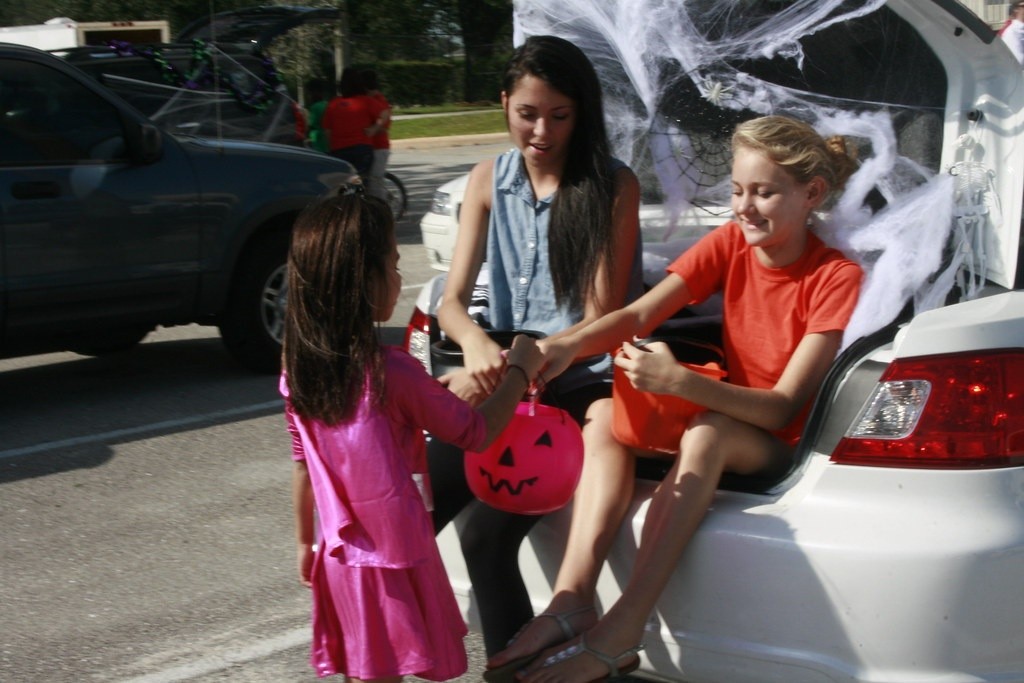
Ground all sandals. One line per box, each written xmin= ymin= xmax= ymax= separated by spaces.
xmin=488 ymin=600 xmax=649 ymax=683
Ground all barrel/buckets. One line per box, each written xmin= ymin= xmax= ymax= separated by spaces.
xmin=608 ymin=337 xmax=729 ymax=458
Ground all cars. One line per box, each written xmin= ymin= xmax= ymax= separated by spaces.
xmin=0 ymin=39 xmax=368 ymax=365
xmin=398 ymin=0 xmax=1021 ymax=683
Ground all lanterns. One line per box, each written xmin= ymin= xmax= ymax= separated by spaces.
xmin=462 ymin=348 xmax=585 ymax=516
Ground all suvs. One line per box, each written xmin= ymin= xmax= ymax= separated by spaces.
xmin=44 ymin=4 xmax=351 ymax=154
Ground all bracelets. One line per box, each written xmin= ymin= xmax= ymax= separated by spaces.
xmin=506 ymin=363 xmax=531 ymax=392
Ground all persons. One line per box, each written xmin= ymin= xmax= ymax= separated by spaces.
xmin=276 ymin=182 xmax=546 ymax=683
xmin=321 ymin=62 xmax=393 ymax=206
xmin=300 ymin=77 xmax=337 ymax=157
xmin=409 ymin=34 xmax=647 ymax=681
xmin=483 ymin=114 xmax=869 ymax=682
